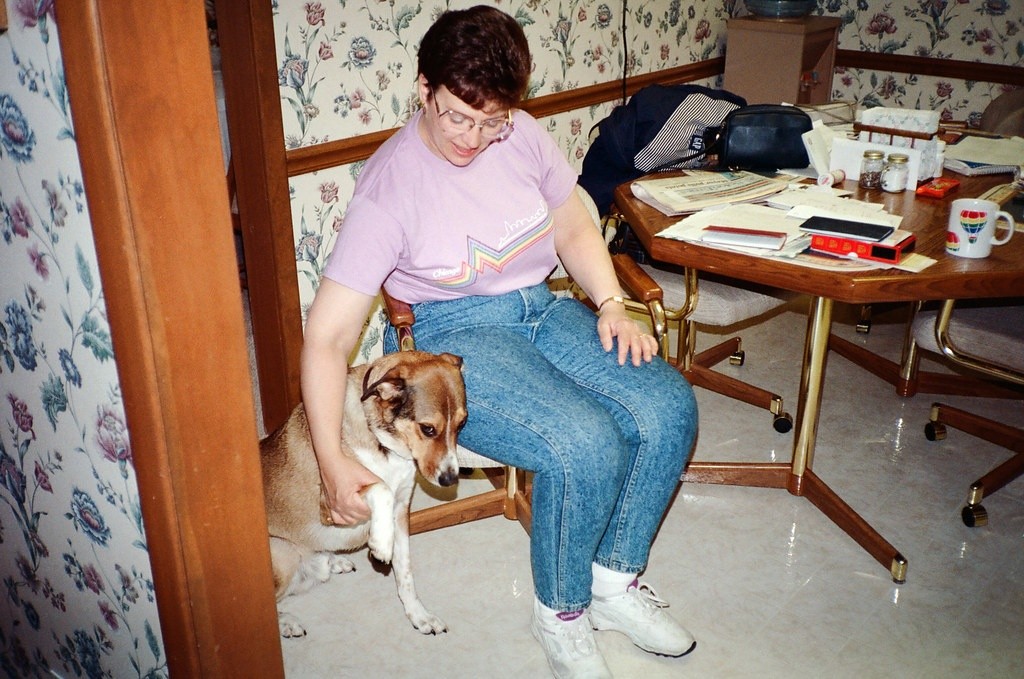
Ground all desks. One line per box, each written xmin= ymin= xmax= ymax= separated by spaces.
xmin=615 ymin=116 xmax=1024 ymax=584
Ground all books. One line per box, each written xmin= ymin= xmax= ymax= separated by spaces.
xmin=703 ymin=226 xmax=787 ymax=249
xmin=800 ymin=216 xmax=895 ymax=241
xmin=943 ymin=147 xmax=1017 ymax=175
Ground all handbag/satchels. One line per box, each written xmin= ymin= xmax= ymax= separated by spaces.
xmin=716 ymin=102 xmax=813 ymax=169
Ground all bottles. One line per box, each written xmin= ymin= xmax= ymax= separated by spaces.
xmin=744 ymin=0 xmax=817 ymax=19
xmin=858 ymin=150 xmax=909 ymax=194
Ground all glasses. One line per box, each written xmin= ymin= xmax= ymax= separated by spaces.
xmin=431 ymin=85 xmax=515 ymax=141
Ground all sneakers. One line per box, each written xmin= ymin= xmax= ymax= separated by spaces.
xmin=588 ymin=579 xmax=697 ymax=657
xmin=531 ymin=594 xmax=612 ymax=679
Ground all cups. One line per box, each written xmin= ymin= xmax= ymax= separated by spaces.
xmin=944 ymin=198 xmax=1015 ymax=259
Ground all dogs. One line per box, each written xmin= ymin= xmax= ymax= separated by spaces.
xmin=256 ymin=351 xmax=468 ymax=642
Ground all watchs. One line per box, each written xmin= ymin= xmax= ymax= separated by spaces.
xmin=597 ymin=296 xmax=624 ymax=312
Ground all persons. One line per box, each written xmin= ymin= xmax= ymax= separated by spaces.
xmin=299 ymin=4 xmax=698 ymax=679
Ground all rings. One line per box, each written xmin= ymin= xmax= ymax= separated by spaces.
xmin=640 ymin=333 xmax=645 ymax=337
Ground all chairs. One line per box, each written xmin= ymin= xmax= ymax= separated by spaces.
xmin=381 ymin=92 xmax=1024 ymax=537
